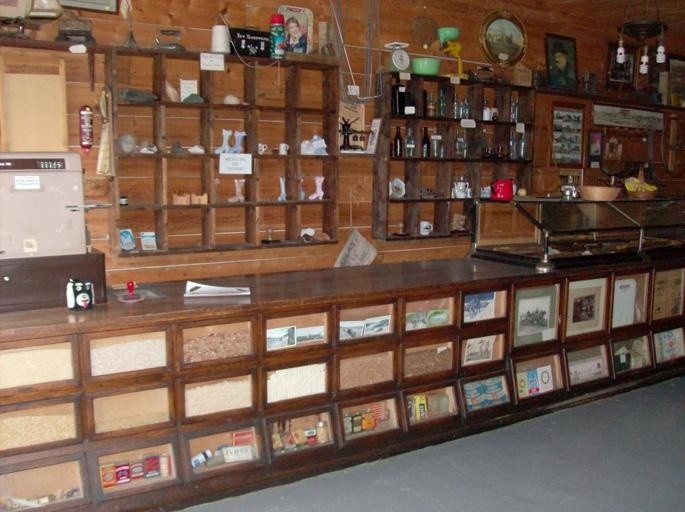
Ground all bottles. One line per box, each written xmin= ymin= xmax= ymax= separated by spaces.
xmin=456 ymin=138 xmax=463 ymax=158
xmin=484 ymin=100 xmax=490 ymax=120
xmin=519 ymin=133 xmax=527 ymax=158
xmin=438 ymin=89 xmax=446 ymax=118
xmin=427 ymin=91 xmax=436 ymax=117
xmin=510 ymin=126 xmax=517 ymax=160
xmin=405 ymin=128 xmax=416 ymax=159
xmin=422 ymin=127 xmax=429 ymax=158
xmin=431 ymin=129 xmax=441 ymax=158
xmin=394 ymin=126 xmax=403 ymax=157
xmin=464 ymin=144 xmax=469 ymax=158
xmin=492 ymin=112 xmax=498 ymax=120
xmin=511 ymin=97 xmax=517 ymax=122
xmin=483 ymin=128 xmax=487 ymax=152
xmin=211 ymin=2 xmax=230 ymax=53
xmin=270 ymin=14 xmax=285 ymax=58
xmin=453 ymin=93 xmax=460 ymax=119
xmin=460 ymin=96 xmax=472 ymax=119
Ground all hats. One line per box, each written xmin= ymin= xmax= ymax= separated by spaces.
xmin=551 ymin=42 xmax=568 ymax=55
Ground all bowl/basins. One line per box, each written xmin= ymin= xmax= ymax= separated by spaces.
xmin=412 ymin=58 xmax=440 ymax=74
xmin=626 ymin=192 xmax=657 ymax=200
xmin=579 ymin=186 xmax=620 ymax=201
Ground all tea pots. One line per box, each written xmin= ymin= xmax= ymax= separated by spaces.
xmin=454 ymin=176 xmax=469 ymax=198
xmin=492 ymin=179 xmax=513 ymax=201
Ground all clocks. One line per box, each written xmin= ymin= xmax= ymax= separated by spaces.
xmin=390 ymin=47 xmax=410 ymax=72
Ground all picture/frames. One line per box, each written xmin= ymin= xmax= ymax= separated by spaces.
xmin=61 ymin=0 xmax=118 ymax=14
xmin=278 ymin=4 xmax=314 ymax=55
xmin=478 ymin=9 xmax=529 ymax=68
xmin=546 ymin=100 xmax=585 ymax=169
xmin=666 ymin=53 xmax=685 ymax=110
xmin=604 ymin=38 xmax=637 ymax=90
xmin=0 ymin=0 xmax=25 ymax=19
xmin=544 ymin=32 xmax=578 ymax=91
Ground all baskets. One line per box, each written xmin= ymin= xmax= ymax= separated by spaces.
xmin=626 ymin=163 xmax=656 ymax=201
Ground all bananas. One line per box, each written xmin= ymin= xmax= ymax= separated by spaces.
xmin=623 ymin=176 xmax=657 ymax=192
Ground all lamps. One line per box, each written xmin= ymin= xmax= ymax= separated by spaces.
xmin=488 ymin=177 xmax=555 ymax=275
xmin=615 ymin=0 xmax=668 ymax=74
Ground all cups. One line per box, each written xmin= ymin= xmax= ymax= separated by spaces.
xmin=316 ymin=421 xmax=329 ymax=442
xmin=420 ymin=220 xmax=433 ymax=235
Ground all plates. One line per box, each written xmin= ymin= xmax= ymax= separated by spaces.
xmin=191 ymin=449 xmax=212 ymax=468
xmin=262 ymin=239 xmax=280 ymax=244
xmin=393 ymin=233 xmax=408 ymax=235
xmin=118 ymin=295 xmax=146 ymax=302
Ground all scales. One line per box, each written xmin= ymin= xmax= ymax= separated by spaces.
xmin=384 ymin=41 xmax=410 ymax=72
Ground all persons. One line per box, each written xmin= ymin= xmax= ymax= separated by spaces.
xmin=284 ymin=17 xmax=306 ymax=53
xmin=550 ymin=41 xmax=576 ymax=90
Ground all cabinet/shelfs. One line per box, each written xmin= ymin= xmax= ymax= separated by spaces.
xmin=0 ymin=38 xmax=340 ymax=259
xmin=372 ymin=70 xmax=536 ymax=240
xmin=470 ymin=197 xmax=685 ymax=267
xmin=1 ymin=237 xmax=685 ymax=512
